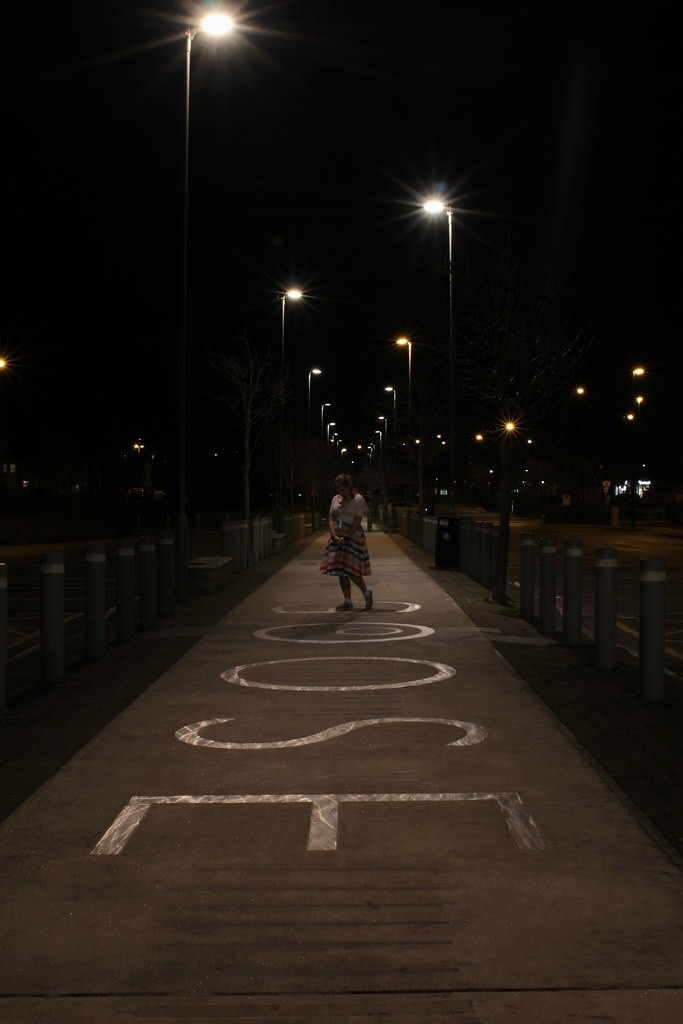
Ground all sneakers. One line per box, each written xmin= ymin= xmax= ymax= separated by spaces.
xmin=365 ymin=590 xmax=374 ymax=608
xmin=335 ymin=601 xmax=354 ymax=611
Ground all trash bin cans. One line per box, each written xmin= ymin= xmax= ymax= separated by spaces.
xmin=399 ymin=512 xmax=408 ymax=535
xmin=435 ymin=516 xmax=473 ymax=570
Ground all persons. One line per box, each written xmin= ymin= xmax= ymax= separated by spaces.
xmin=318 ymin=474 xmax=374 ymax=611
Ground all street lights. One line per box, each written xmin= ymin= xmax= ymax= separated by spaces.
xmin=325 ymin=421 xmax=346 ymax=465
xmin=421 ymin=199 xmax=458 ymax=515
xmin=306 ymin=368 xmax=322 ymax=533
xmin=175 ymin=10 xmax=239 ymax=602
xmin=397 ymin=336 xmax=411 ymax=505
xmin=273 ymin=289 xmax=304 ymax=532
xmin=374 ymin=429 xmax=383 ymax=468
xmin=384 ymin=386 xmax=396 ymax=434
xmin=378 ymin=416 xmax=388 ymax=444
